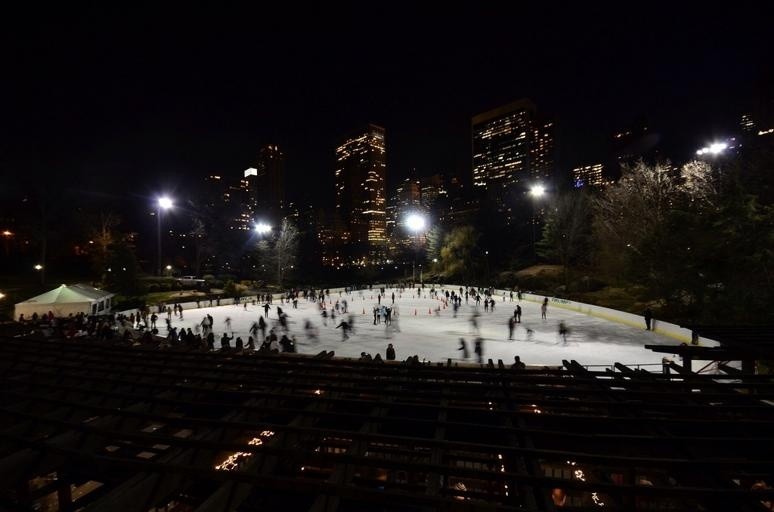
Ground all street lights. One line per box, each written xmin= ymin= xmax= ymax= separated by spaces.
xmin=157 ymin=194 xmax=173 ymax=276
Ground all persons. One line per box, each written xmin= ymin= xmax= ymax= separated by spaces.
xmin=645 ymin=308 xmax=652 ymax=330
xmin=20 ymin=280 xmax=568 ymax=372
